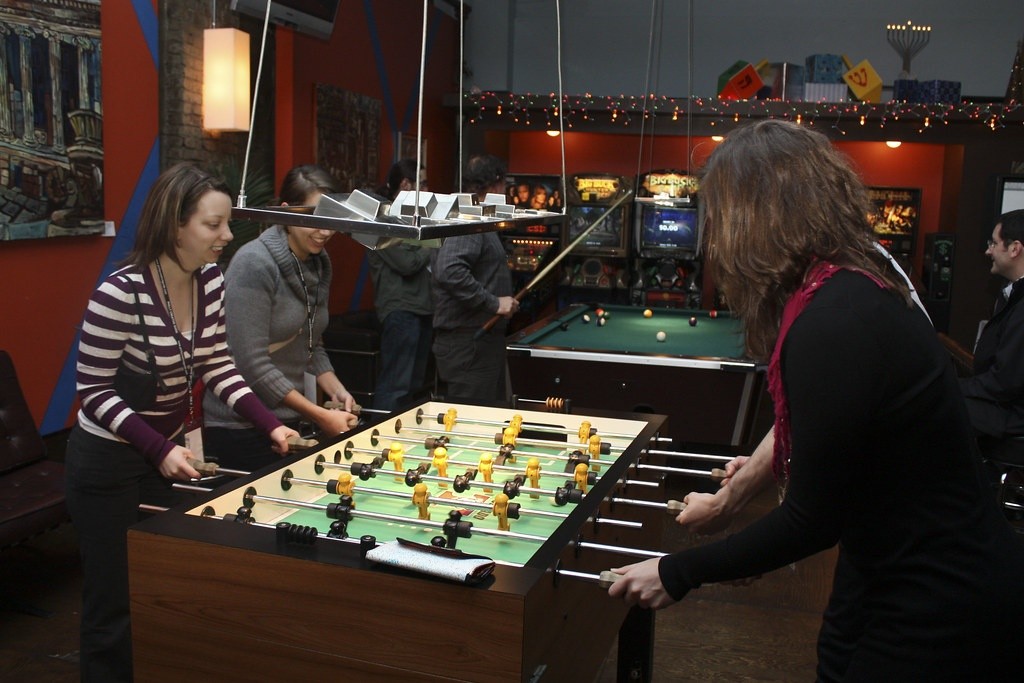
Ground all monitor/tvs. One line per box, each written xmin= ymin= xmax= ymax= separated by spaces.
xmin=231 ymin=0 xmax=341 ymax=41
xmin=636 ymin=198 xmax=706 ymax=258
xmin=562 ymin=201 xmax=630 ymax=257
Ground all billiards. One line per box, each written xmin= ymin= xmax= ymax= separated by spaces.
xmin=643 ymin=309 xmax=653 ymax=318
xmin=581 ymin=312 xmax=591 ymax=323
xmin=689 ymin=317 xmax=697 ymax=326
xmin=656 ymin=331 xmax=666 ymax=341
xmin=595 ymin=308 xmax=611 ymax=327
xmin=709 ymin=310 xmax=718 ymax=318
xmin=560 ymin=322 xmax=569 ymax=331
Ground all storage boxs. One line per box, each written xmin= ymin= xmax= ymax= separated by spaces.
xmin=893 ymin=80 xmax=962 ymax=104
xmin=758 ymin=53 xmax=848 ymax=102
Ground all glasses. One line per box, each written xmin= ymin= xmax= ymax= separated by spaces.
xmin=987 ymin=240 xmax=1003 ymax=249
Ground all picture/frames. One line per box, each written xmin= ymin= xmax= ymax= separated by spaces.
xmin=397 ymin=132 xmax=428 ymax=172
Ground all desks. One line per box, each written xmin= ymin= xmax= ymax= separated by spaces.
xmin=503 ymin=300 xmax=770 ymax=447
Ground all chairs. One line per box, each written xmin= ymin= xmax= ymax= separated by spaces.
xmin=0 ymin=350 xmax=73 ymax=620
xmin=321 ymin=309 xmax=383 ymax=424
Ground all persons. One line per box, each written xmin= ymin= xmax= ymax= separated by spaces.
xmin=609 ymin=120 xmax=1024 ymax=683
xmin=64 ymin=161 xmax=299 ymax=683
xmin=367 ymin=158 xmax=434 ymax=416
xmin=959 ymin=209 xmax=1024 ymax=514
xmin=436 ymin=159 xmax=519 ymax=409
xmin=203 ymin=162 xmax=359 ymax=473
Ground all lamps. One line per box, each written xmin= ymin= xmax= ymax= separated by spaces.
xmin=885 ymin=18 xmax=931 ymax=72
xmin=203 ymin=28 xmax=251 ymax=132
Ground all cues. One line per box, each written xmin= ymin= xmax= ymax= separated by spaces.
xmin=475 ymin=188 xmax=634 ymax=340
